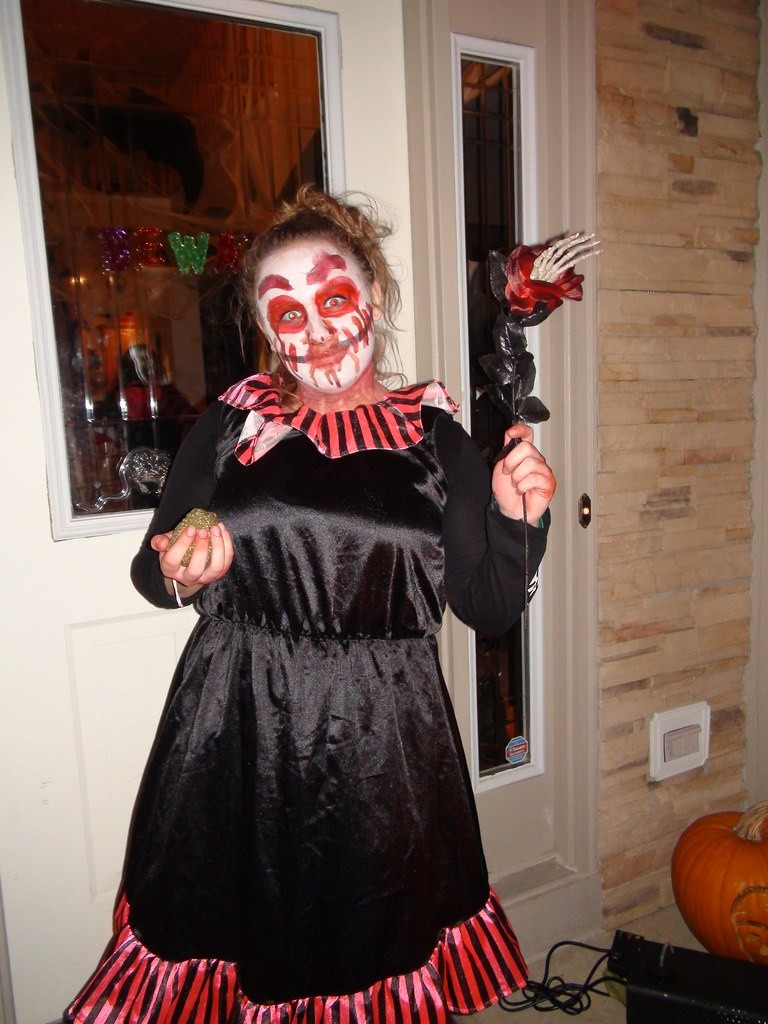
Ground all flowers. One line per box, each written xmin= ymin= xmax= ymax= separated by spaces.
xmin=479 ymin=242 xmax=587 ymax=631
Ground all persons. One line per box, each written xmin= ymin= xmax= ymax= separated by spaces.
xmin=51 ymin=186 xmax=557 ymax=1024
